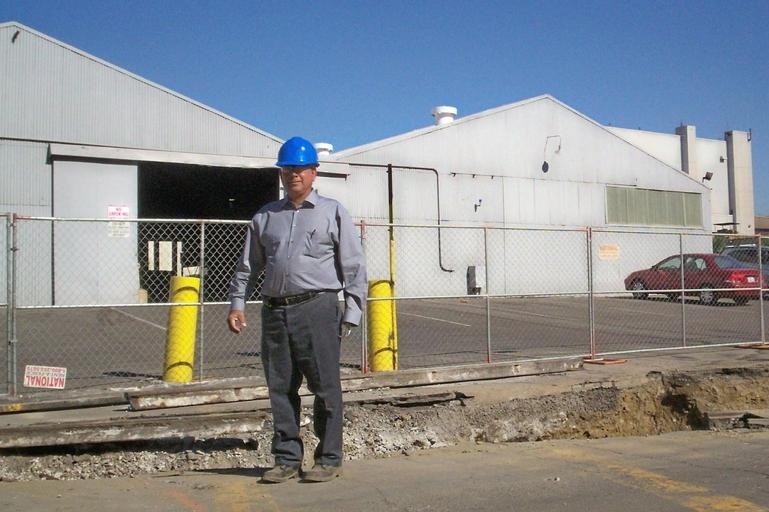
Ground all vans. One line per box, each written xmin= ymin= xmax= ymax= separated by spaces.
xmin=719 ymin=246 xmax=769 ymax=283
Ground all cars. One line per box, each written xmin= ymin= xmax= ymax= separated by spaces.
xmin=624 ymin=253 xmax=768 ymax=305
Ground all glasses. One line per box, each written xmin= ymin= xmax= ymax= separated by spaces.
xmin=281 ymin=166 xmax=305 ymax=172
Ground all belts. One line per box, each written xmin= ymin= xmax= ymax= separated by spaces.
xmin=262 ymin=290 xmax=324 ymax=307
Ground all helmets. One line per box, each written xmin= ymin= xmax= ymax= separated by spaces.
xmin=275 ymin=136 xmax=319 ymax=166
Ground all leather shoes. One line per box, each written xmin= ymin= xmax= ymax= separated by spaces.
xmin=262 ymin=464 xmax=301 ymax=482
xmin=302 ymin=464 xmax=343 ymax=481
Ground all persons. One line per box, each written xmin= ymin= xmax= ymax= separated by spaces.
xmin=226 ymin=135 xmax=369 ymax=484
